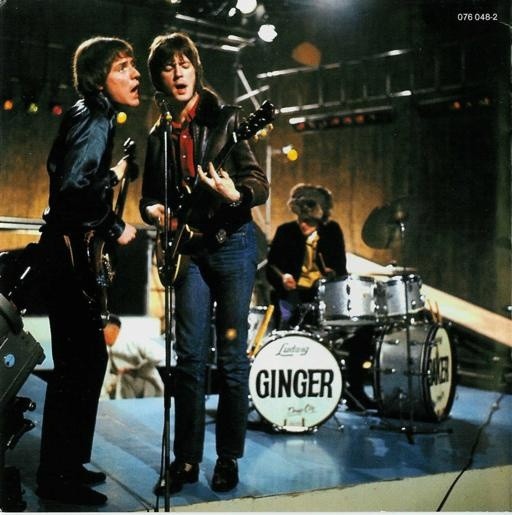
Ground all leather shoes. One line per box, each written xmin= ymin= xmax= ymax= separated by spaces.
xmin=212 ymin=458 xmax=239 ymax=492
xmin=345 ymin=387 xmax=379 ymax=409
xmin=153 ymin=461 xmax=199 ymax=496
xmin=36 ymin=463 xmax=108 ymax=505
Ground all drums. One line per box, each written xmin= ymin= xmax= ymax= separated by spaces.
xmin=317 ymin=273 xmax=377 ymax=326
xmin=211 ymin=305 xmax=268 ymax=367
xmin=362 ymin=264 xmax=425 ymax=325
xmin=371 ymin=318 xmax=456 ymax=422
xmin=248 ymin=325 xmax=344 ymax=433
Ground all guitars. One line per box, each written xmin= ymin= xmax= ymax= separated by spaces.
xmin=82 ymin=137 xmax=135 ymax=307
xmin=154 ymin=95 xmax=281 ymax=288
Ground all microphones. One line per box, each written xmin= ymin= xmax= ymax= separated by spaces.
xmin=154 ymin=91 xmax=173 ymax=122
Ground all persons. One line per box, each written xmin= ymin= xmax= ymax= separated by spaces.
xmin=139 ymin=31 xmax=269 ymax=495
xmin=36 ymin=37 xmax=140 ymax=506
xmin=268 ymin=181 xmax=346 ymax=328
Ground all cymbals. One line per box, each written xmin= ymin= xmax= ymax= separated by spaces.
xmin=361 ymin=194 xmax=456 ymax=249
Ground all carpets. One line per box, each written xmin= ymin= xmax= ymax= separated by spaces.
xmin=17 ymin=373 xmax=512 ymax=511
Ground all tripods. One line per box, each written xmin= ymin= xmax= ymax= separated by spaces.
xmin=370 ymin=225 xmax=453 ymax=445
